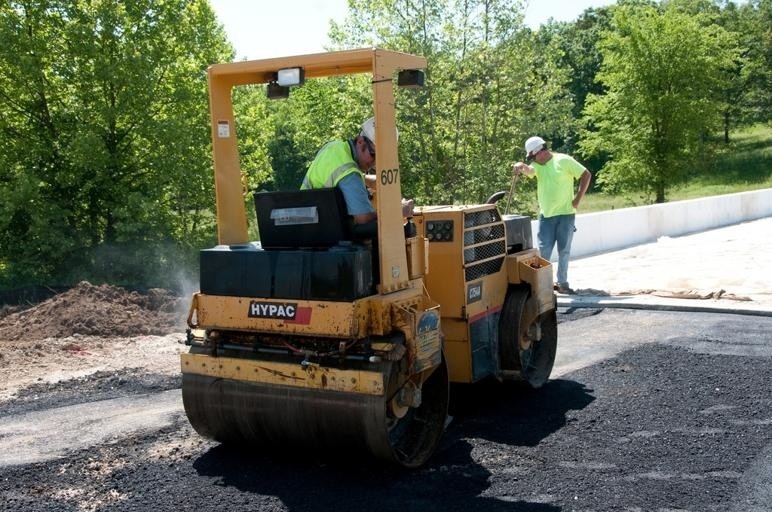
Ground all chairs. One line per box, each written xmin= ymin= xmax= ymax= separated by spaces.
xmin=253 ymin=187 xmax=376 ymax=250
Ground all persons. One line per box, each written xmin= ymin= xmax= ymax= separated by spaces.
xmin=300 ymin=116 xmax=415 ymax=226
xmin=513 ymin=135 xmax=593 ymax=296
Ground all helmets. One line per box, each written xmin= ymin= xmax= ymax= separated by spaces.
xmin=524 ymin=137 xmax=544 ymax=158
xmin=360 ymin=118 xmax=399 ymax=151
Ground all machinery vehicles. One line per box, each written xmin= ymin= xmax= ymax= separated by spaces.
xmin=180 ymin=49 xmax=559 ymax=470
